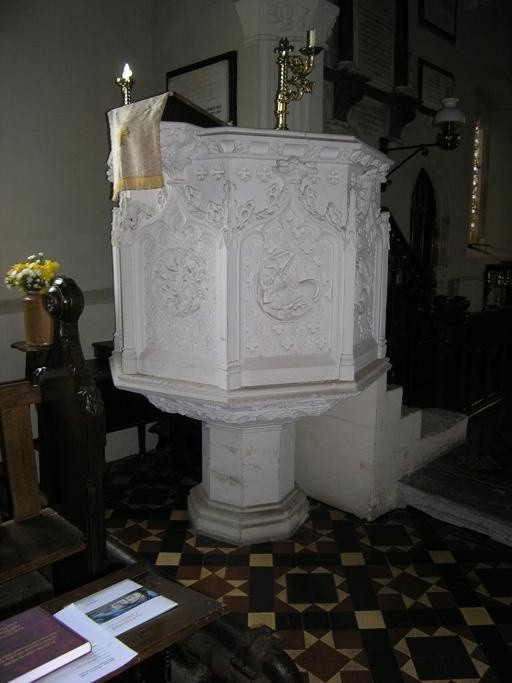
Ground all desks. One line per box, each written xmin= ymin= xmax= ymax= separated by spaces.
xmin=0 ymin=558 xmax=224 ymax=683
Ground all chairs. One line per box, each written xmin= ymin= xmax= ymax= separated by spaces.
xmin=0 ymin=380 xmax=86 ymax=603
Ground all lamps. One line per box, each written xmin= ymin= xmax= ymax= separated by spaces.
xmin=377 ymin=86 xmax=467 ymax=162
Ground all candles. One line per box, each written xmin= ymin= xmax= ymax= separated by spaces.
xmin=309 ymin=28 xmax=316 ymax=48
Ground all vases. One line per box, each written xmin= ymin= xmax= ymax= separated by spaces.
xmin=23 ymin=289 xmax=56 ymax=347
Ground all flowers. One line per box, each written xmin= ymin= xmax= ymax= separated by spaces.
xmin=4 ymin=253 xmax=66 ymax=295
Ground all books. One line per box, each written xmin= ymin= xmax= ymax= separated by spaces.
xmin=0 ymin=606 xmax=93 ymax=683
